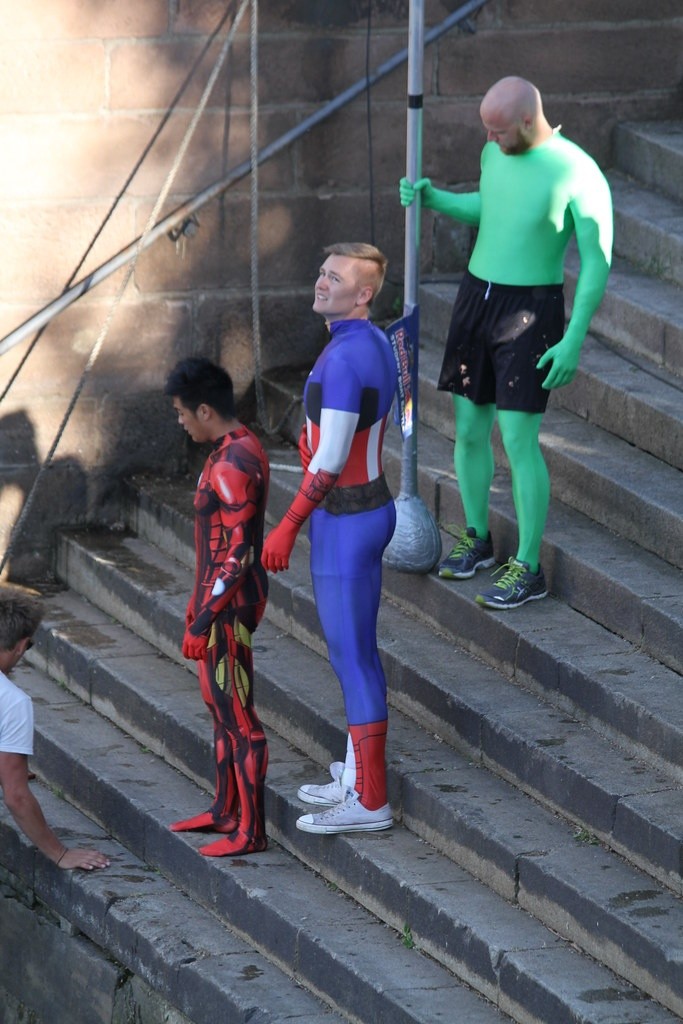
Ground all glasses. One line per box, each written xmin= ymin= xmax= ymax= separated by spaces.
xmin=12 ymin=637 xmax=35 ymax=649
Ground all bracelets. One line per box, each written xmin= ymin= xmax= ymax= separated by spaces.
xmin=56 ymin=847 xmax=68 ymax=865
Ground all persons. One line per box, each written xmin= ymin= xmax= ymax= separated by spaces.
xmin=399 ymin=75 xmax=614 ymax=609
xmin=257 ymin=242 xmax=399 ymax=834
xmin=165 ymin=349 xmax=272 ymax=859
xmin=0 ymin=584 xmax=111 ymax=871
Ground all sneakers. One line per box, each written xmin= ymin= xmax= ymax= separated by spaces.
xmin=475 ymin=555 xmax=549 ymax=609
xmin=439 ymin=525 xmax=495 ymax=579
xmin=296 ymin=787 xmax=394 ymax=834
xmin=297 ymin=762 xmax=345 ymax=807
